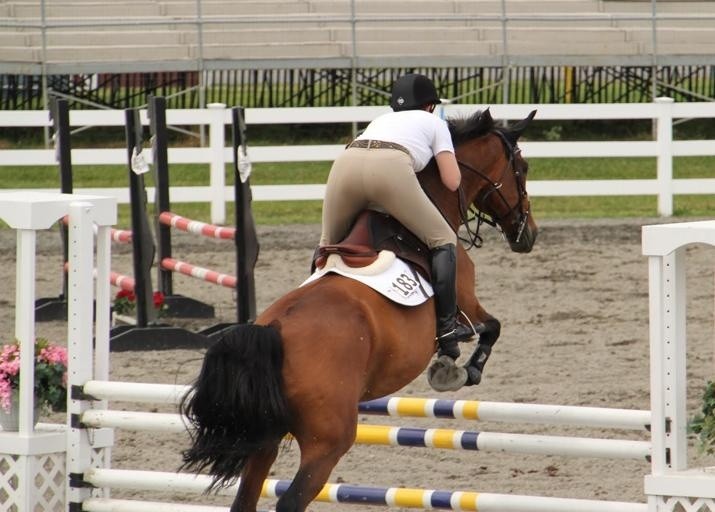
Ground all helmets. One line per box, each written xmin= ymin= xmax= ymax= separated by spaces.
xmin=390 ymin=72 xmax=441 ymax=113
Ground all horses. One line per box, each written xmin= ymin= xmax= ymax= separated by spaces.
xmin=175 ymin=105 xmax=539 ymax=512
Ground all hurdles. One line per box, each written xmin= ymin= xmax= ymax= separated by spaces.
xmin=147 ymin=97 xmax=260 ymax=352
xmin=45 ymin=99 xmax=158 ymax=326
xmin=0 ymin=193 xmax=715 ymax=511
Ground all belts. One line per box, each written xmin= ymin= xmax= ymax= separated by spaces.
xmin=344 ymin=137 xmax=409 ymax=158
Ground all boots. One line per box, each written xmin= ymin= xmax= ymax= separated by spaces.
xmin=428 ymin=244 xmax=485 ymax=345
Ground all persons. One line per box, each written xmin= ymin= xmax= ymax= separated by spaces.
xmin=311 ymin=72 xmax=487 ymax=357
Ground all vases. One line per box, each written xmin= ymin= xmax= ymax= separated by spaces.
xmin=112 ymin=312 xmax=136 ymax=326
xmin=0 ymin=389 xmax=41 ymax=432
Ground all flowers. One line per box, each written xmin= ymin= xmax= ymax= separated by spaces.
xmin=113 ymin=290 xmax=164 ymax=320
xmin=0 ymin=337 xmax=68 ymax=417
